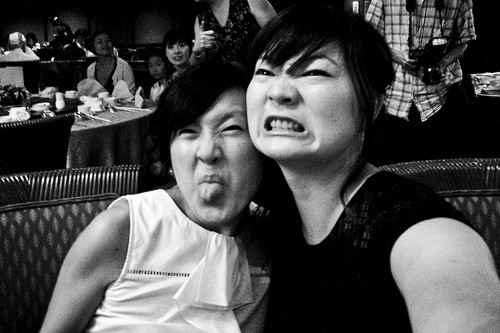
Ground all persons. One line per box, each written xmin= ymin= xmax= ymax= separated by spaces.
xmin=39 ymin=59 xmax=274 ymax=333
xmin=188 ymin=0 xmax=278 ymax=67
xmin=87 ymin=30 xmax=136 ymax=96
xmin=148 ymin=51 xmax=171 ymax=104
xmin=365 ymin=0 xmax=477 ymax=164
xmin=163 ymin=26 xmax=192 ymax=81
xmin=0 ymin=29 xmax=118 ymax=88
xmin=246 ymin=0 xmax=500 ymax=333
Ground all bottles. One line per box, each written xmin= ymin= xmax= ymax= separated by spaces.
xmin=56 ymin=92 xmax=65 ymax=109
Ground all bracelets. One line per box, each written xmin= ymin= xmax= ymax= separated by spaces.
xmin=193 ymin=53 xmax=202 ymax=60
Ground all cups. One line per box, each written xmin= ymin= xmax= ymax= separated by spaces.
xmin=10 ymin=108 xmax=26 ymax=118
xmin=33 ymin=102 xmax=50 ymax=109
xmin=98 ymin=93 xmax=109 ymax=98
xmin=66 ymin=91 xmax=76 ymax=97
xmin=106 ymin=97 xmax=117 ymax=106
xmin=78 ymin=106 xmax=90 ymax=114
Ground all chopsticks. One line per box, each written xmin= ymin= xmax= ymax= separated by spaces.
xmin=86 ymin=114 xmax=110 ymax=123
xmin=116 ymin=107 xmax=151 ymax=111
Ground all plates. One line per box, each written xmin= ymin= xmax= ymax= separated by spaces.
xmin=0 ymin=113 xmax=30 ymax=123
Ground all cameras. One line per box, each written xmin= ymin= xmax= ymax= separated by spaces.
xmin=409 ymin=35 xmax=454 ymax=85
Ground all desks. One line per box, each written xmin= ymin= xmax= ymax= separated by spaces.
xmin=0 ymin=98 xmax=157 ymax=172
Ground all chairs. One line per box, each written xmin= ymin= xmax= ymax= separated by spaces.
xmin=0 ymin=61 xmax=500 ymax=333
